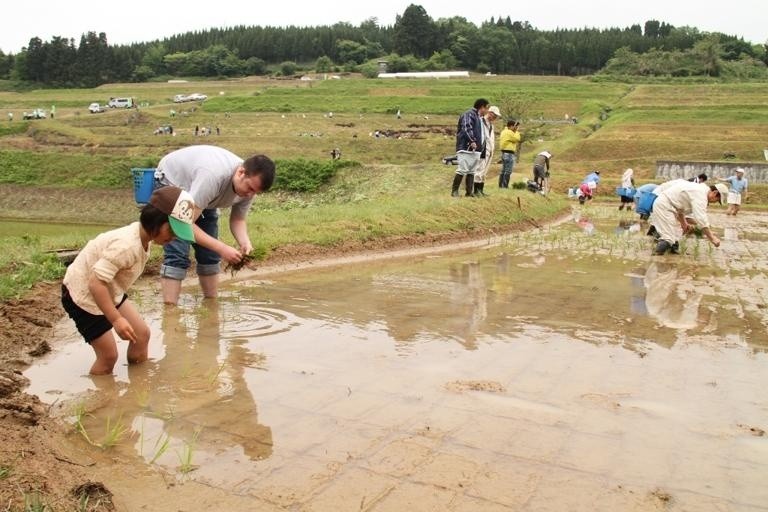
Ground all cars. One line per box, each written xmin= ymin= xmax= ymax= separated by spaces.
xmin=443 ymin=155 xmax=459 ymax=165
xmin=176 ymin=93 xmax=207 ymax=102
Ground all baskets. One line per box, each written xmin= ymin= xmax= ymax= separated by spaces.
xmin=131 ymin=168 xmax=156 ymax=203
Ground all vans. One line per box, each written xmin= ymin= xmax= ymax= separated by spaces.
xmin=108 ymin=97 xmax=133 ymax=109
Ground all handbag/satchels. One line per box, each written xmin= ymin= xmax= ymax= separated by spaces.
xmin=636 ymin=191 xmax=658 ymax=215
xmin=617 ymin=186 xmax=636 ymax=199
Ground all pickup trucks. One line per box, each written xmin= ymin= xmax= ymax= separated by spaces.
xmin=88 ymin=103 xmax=105 ymax=113
xmin=23 ymin=108 xmax=46 ymax=120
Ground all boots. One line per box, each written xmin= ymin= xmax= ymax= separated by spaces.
xmin=465 ymin=174 xmax=475 ymax=197
xmin=480 ymin=182 xmax=490 ymax=196
xmin=502 ymin=174 xmax=510 ymax=187
xmin=474 ymin=183 xmax=483 ymax=197
xmin=671 ymin=241 xmax=680 ymax=255
xmin=451 ymin=174 xmax=462 ymax=197
xmin=654 ymin=240 xmax=671 ymax=255
xmin=499 ymin=174 xmax=503 ymax=187
xmin=647 ymin=225 xmax=655 ymax=235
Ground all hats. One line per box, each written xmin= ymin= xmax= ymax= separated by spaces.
xmin=487 ymin=105 xmax=501 ymax=116
xmin=736 ymin=168 xmax=744 ymax=174
xmin=148 ymin=186 xmax=196 ymax=245
xmin=715 ymin=183 xmax=729 ymax=207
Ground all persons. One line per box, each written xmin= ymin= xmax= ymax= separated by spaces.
xmin=60 ymin=187 xmax=196 ymax=375
xmin=576 ymin=182 xmax=596 ymax=205
xmin=7 ymin=104 xmax=57 ymax=120
xmin=153 ymin=144 xmax=276 ymax=305
xmin=153 ymin=124 xmax=173 ymax=135
xmin=473 ymin=106 xmax=502 ymax=197
xmin=716 ymin=168 xmax=748 ymax=217
xmin=330 ymin=148 xmax=341 ymax=161
xmin=581 ymin=170 xmax=601 ymax=192
xmin=452 ymin=98 xmax=489 ymax=196
xmin=619 ymin=168 xmax=729 ymax=257
xmin=497 ymin=120 xmax=521 ymax=188
xmin=193 ymin=125 xmax=220 ymax=136
xmin=534 ymin=150 xmax=552 ymax=192
